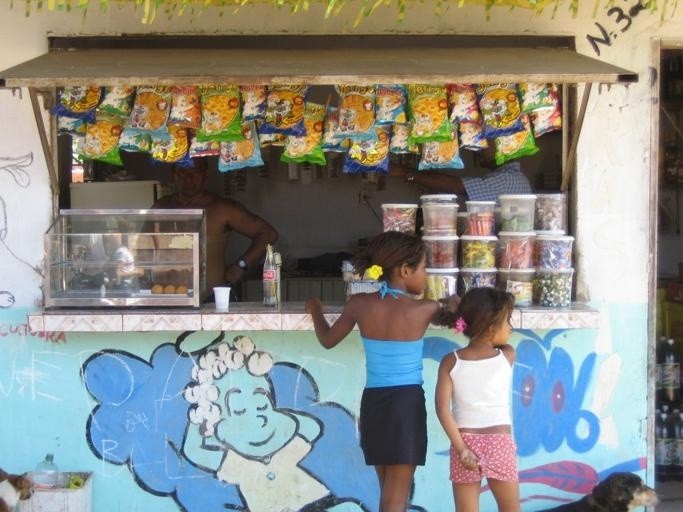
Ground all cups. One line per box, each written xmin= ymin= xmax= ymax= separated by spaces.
xmin=212 ymin=287 xmax=232 ymax=313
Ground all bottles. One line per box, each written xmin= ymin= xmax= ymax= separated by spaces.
xmin=31 ymin=453 xmax=58 ymax=489
xmin=662 ymin=339 xmax=681 ymax=406
xmin=653 ymin=405 xmax=682 ymax=484
xmin=655 ymin=336 xmax=665 ymax=406
xmin=261 ymin=244 xmax=278 ymax=308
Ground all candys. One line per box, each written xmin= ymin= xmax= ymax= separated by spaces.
xmin=499 ymin=236 xmax=532 ymax=270
xmin=457 ymin=272 xmax=497 ymax=299
xmin=381 ymin=208 xmax=417 ymax=233
xmin=534 ymin=236 xmax=574 ymax=271
xmin=498 ymin=273 xmax=533 ymax=308
xmin=533 ymin=270 xmax=573 ymax=309
xmin=534 ymin=194 xmax=569 ymax=233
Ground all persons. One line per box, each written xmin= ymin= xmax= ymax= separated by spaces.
xmin=382 ymin=137 xmax=532 ymax=235
xmin=133 ymin=156 xmax=277 ymax=301
xmin=431 ymin=286 xmax=522 ymax=512
xmin=304 ymin=231 xmax=460 ymax=512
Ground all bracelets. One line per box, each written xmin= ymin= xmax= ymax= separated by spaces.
xmin=404 ymin=168 xmax=415 ymax=183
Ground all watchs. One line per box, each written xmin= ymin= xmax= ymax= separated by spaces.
xmin=236 ymin=258 xmax=248 ymax=271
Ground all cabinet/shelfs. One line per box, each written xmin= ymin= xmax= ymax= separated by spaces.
xmin=40 ymin=205 xmax=209 ymax=309
xmin=237 ymin=274 xmax=347 ymax=303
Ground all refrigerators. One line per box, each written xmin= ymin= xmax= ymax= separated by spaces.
xmin=69 ymin=179 xmax=171 ymax=275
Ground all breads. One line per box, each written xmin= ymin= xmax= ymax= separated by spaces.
xmin=150 ymin=284 xmax=164 ymax=294
xmin=164 ymin=284 xmax=177 ymax=294
xmin=176 ymin=285 xmax=188 ymax=294
